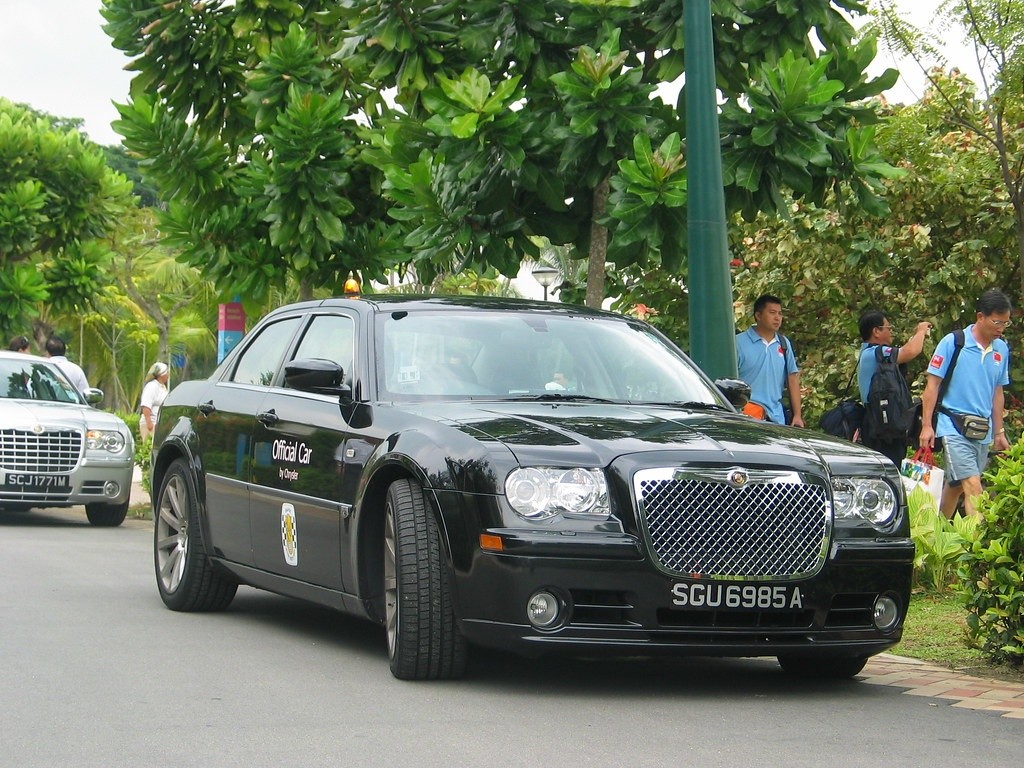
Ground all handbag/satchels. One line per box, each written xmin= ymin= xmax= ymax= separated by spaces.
xmin=782 ymin=405 xmax=790 ymax=425
xmin=912 ymin=400 xmax=944 ymax=452
xmin=818 ymin=399 xmax=865 ymax=438
xmin=937 ymin=405 xmax=990 ymax=440
xmin=901 ymin=446 xmax=944 ymax=517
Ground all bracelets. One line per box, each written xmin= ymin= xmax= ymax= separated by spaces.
xmin=994 ymin=428 xmax=1005 ymax=436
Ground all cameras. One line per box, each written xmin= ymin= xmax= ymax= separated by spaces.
xmin=924 ymin=325 xmax=930 ymax=339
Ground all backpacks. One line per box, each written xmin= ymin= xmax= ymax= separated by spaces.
xmin=863 ymin=344 xmax=917 ymax=449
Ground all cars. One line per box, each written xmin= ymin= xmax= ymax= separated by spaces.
xmin=149 ymin=292 xmax=917 ymax=680
xmin=0 ymin=350 xmax=136 ymax=527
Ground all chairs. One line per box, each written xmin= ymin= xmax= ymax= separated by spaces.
xmin=419 ymin=356 xmax=478 ymax=395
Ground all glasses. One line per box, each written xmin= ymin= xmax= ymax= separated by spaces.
xmin=877 ymin=325 xmax=895 ymax=329
xmin=984 ymin=316 xmax=1012 ymax=327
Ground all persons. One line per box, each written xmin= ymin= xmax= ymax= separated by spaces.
xmin=735 ymin=295 xmax=804 ymax=428
xmin=919 ymin=291 xmax=1010 ymax=522
xmin=9 ymin=336 xmax=29 ymax=354
xmin=857 ymin=311 xmax=932 ymax=472
xmin=45 ymin=336 xmax=96 ymax=407
xmin=139 ymin=363 xmax=169 ymax=465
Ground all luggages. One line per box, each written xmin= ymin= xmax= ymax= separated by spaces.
xmin=957 ymin=451 xmax=1010 ymax=518
